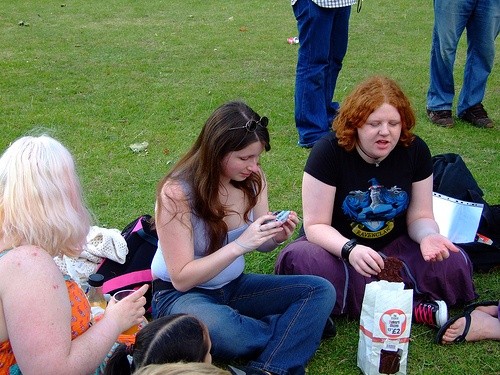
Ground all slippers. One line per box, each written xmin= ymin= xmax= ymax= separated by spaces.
xmin=436 ymin=307 xmax=475 ymax=346
xmin=463 ymin=300 xmax=499 ymax=319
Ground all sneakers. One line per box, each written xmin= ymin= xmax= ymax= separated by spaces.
xmin=458 ymin=103 xmax=494 ymax=128
xmin=412 ymin=300 xmax=448 ymax=328
xmin=426 ymin=107 xmax=454 ymax=127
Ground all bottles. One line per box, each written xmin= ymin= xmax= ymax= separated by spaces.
xmin=85 ymin=273 xmax=106 ymax=320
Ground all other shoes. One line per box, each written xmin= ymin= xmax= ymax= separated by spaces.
xmin=321 ymin=316 xmax=337 ymax=340
xmin=246 ymin=367 xmax=278 ymax=375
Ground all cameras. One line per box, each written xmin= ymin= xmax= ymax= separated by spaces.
xmin=262 ymin=210 xmax=291 ymax=228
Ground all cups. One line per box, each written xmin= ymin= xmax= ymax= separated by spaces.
xmin=114 ymin=290 xmax=139 ymax=335
xmin=287 ymin=37 xmax=299 ymax=43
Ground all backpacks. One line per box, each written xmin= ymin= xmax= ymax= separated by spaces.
xmin=86 ymin=214 xmax=159 ymax=320
xmin=432 ymin=153 xmax=500 ymax=273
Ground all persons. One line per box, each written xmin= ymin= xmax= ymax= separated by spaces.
xmin=103 ymin=312 xmax=233 ymax=375
xmin=292 ymin=0 xmax=362 ymax=148
xmin=426 ymin=0 xmax=500 ymax=129
xmin=148 ymin=102 xmax=339 ymax=375
xmin=435 ymin=298 xmax=499 ymax=342
xmin=0 ymin=133 xmax=150 ymax=375
xmin=275 ymin=76 xmax=477 ymax=326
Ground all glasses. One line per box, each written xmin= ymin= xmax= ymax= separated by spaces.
xmin=227 ymin=116 xmax=269 ymax=132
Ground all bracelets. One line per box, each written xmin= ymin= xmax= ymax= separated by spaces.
xmin=341 ymin=239 xmax=356 ymax=260
